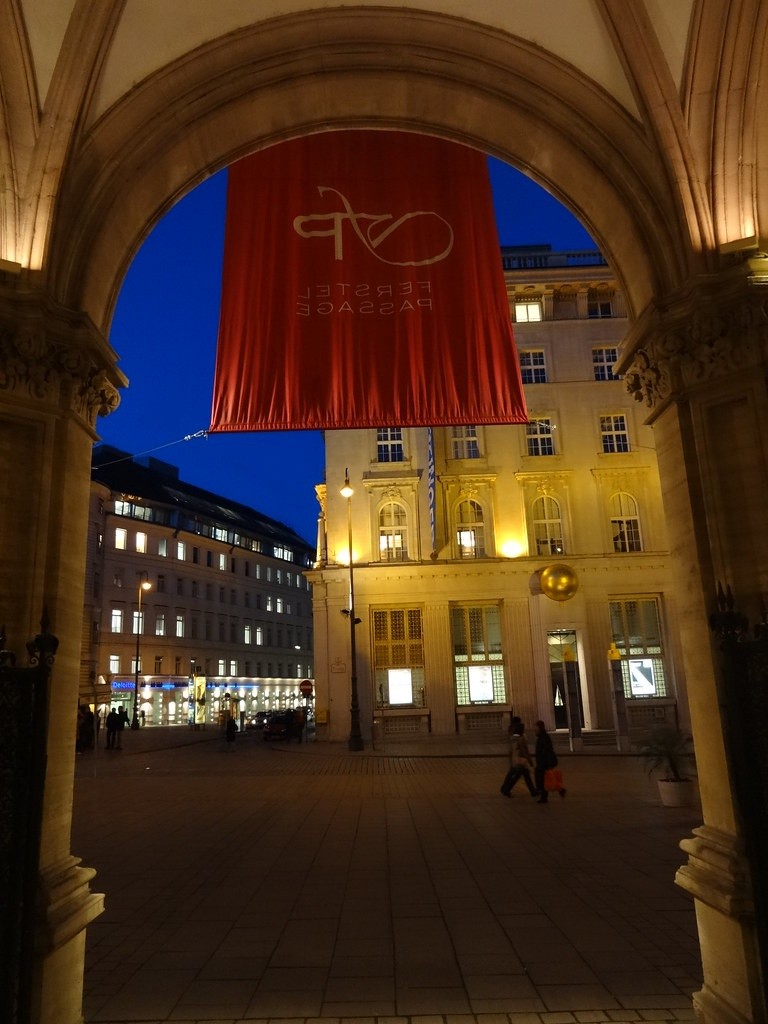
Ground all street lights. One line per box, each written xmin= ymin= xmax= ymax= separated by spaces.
xmin=128 ymin=569 xmax=151 ymax=730
xmin=337 ymin=467 xmax=367 ymax=752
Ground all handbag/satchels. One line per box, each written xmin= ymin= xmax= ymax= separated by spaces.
xmin=544 ymin=768 xmax=567 ymax=792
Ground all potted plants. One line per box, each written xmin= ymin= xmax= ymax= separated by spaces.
xmin=638 ymin=730 xmax=697 ymax=807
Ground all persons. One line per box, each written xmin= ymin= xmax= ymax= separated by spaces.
xmin=500 ymin=716 xmax=536 ymax=797
xmin=124 ymin=708 xmax=131 ymax=727
xmin=534 ymin=720 xmax=566 ymax=803
xmin=295 ymin=707 xmax=305 ymax=743
xmin=106 ymin=706 xmax=125 ymax=750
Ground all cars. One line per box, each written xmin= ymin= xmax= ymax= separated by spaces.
xmin=249 ymin=709 xmax=289 ymax=741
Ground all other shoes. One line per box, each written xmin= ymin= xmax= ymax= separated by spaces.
xmin=536 ymin=796 xmax=549 ymax=804
xmin=530 ymin=790 xmax=539 ymax=797
xmin=500 ymin=784 xmax=514 ymax=799
xmin=104 ymin=745 xmax=123 ymax=752
xmin=559 ymin=789 xmax=567 ymax=799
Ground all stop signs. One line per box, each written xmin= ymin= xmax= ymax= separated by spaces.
xmin=299 ymin=680 xmax=313 ymax=695
